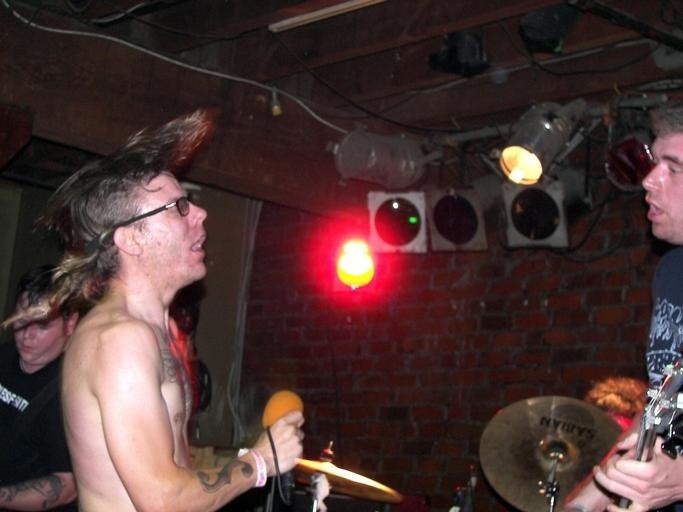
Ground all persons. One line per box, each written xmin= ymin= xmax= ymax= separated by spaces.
xmin=0 ymin=266 xmax=79 ymax=512
xmin=561 ymin=100 xmax=682 ymax=511
xmin=0 ymin=110 xmax=307 ymax=511
xmin=565 ymin=376 xmax=649 ymax=503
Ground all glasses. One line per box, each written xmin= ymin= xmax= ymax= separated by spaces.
xmin=110 ymin=197 xmax=189 ymax=245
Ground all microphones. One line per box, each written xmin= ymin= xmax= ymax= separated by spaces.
xmin=263 ymin=390 xmax=304 ymax=504
xmin=519 ymin=0 xmax=597 ymax=54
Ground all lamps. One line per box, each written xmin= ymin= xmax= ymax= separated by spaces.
xmin=334 ymin=98 xmax=603 ymax=195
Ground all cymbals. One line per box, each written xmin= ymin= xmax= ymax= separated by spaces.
xmin=479 ymin=397 xmax=622 ymax=511
xmin=294 ymin=458 xmax=402 ymax=504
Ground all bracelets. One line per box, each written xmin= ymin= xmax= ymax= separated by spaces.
xmin=250 ymin=449 xmax=268 ymax=487
